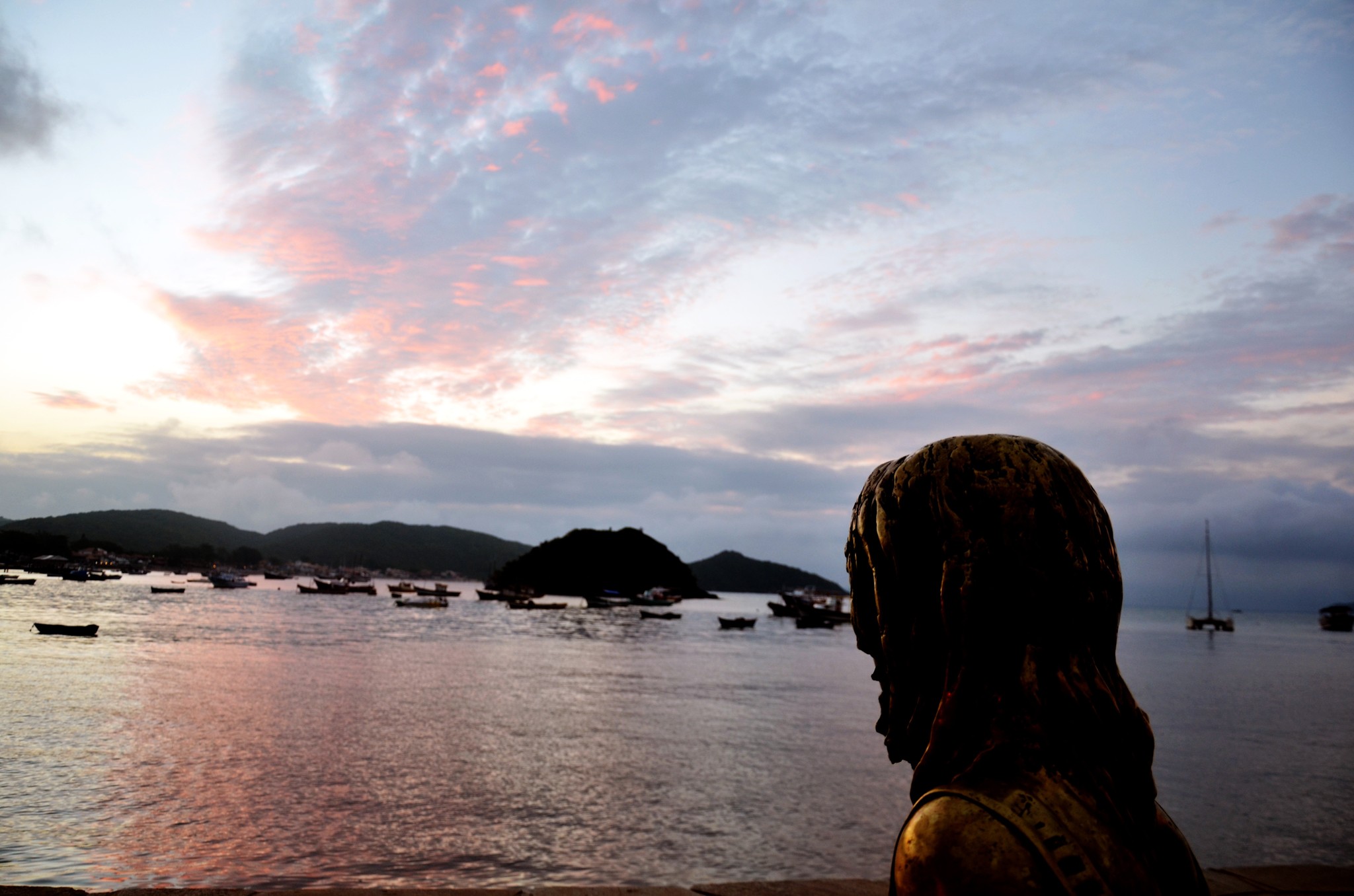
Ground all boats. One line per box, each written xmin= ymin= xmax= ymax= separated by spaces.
xmin=478 ymin=587 xmax=567 ymax=610
xmin=394 ymin=597 xmax=449 ymax=607
xmin=413 ymin=582 xmax=461 ymax=597
xmin=638 ymin=608 xmax=682 ymax=620
xmin=62 ymin=561 xmax=151 ymax=582
xmin=0 ymin=575 xmax=36 ymax=585
xmin=296 ymin=570 xmax=377 ymax=596
xmin=717 ymin=615 xmax=757 ymax=630
xmin=581 ymin=586 xmax=683 ymax=609
xmin=387 ymin=578 xmax=417 ymax=593
xmin=0 ymin=574 xmax=19 ymax=578
xmin=150 ymin=586 xmax=185 ymax=593
xmin=33 ymin=622 xmax=100 ymax=636
xmin=200 ymin=564 xmax=257 ymax=589
xmin=263 ymin=570 xmax=293 ymax=579
xmin=766 ymin=584 xmax=851 ymax=631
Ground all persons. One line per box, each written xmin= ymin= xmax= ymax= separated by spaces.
xmin=845 ymin=434 xmax=1213 ymax=896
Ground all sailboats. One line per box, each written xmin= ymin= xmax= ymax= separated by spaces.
xmin=1184 ymin=520 xmax=1236 ymax=630
